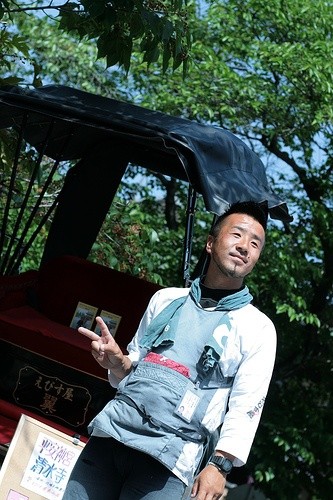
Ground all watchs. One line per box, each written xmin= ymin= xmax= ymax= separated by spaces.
xmin=208 ymin=454 xmax=235 ymax=475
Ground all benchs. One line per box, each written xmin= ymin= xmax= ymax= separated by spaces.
xmin=1 ymin=254 xmax=165 ymax=378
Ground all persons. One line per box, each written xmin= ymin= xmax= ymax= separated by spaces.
xmin=62 ymin=200 xmax=277 ymax=500
